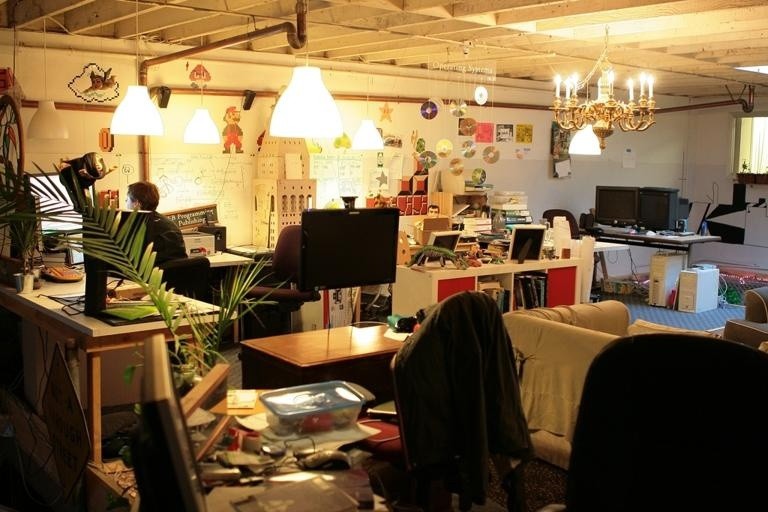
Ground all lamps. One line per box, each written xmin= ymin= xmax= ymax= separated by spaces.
xmin=112 ymin=3 xmax=166 ymax=139
xmin=568 ymin=82 xmax=601 ymax=155
xmin=352 ymin=63 xmax=385 ymax=151
xmin=551 ymin=24 xmax=657 ymax=151
xmin=183 ymin=34 xmax=221 ymax=145
xmin=268 ymin=0 xmax=344 ymax=140
xmin=28 ymin=17 xmax=69 ymax=140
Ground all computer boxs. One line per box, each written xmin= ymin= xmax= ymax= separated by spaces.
xmin=678 ymin=268 xmax=720 ymax=314
xmin=648 ymin=254 xmax=687 ymax=307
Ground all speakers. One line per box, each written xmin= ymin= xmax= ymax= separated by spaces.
xmin=242 ymin=89 xmax=256 ymax=110
xmin=158 ymin=86 xmax=171 ymax=108
xmin=198 ymin=225 xmax=226 ymax=253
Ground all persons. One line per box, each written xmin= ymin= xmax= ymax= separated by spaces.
xmin=426 ymin=205 xmax=439 ymax=218
xmin=122 ymin=181 xmax=187 ymax=261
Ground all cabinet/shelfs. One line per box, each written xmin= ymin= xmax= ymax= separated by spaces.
xmin=391 ymin=256 xmax=580 ymax=317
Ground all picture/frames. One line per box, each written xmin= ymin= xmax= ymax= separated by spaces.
xmin=506 ymin=225 xmax=546 ymax=264
xmin=422 ymin=230 xmax=462 ymax=268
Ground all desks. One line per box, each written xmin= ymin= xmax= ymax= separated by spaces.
xmin=0 ymin=264 xmax=237 ymax=482
xmin=593 ymin=240 xmax=629 ymax=281
xmin=204 ymin=247 xmax=251 ymax=346
xmin=578 ymin=222 xmax=722 ymax=288
xmin=240 ymin=320 xmax=415 ymax=388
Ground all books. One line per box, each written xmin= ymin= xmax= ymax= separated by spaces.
xmin=462 ymin=218 xmax=493 ymax=234
xmin=479 ymin=275 xmax=545 ymax=315
xmin=489 ymin=190 xmax=532 ymax=224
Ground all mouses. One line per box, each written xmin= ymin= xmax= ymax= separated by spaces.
xmin=296 ymin=449 xmax=351 ymax=471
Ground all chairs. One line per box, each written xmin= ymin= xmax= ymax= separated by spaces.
xmin=154 ymin=256 xmax=211 ymax=302
xmin=530 ymin=329 xmax=767 ymax=512
xmin=233 ymin=224 xmax=321 ymax=336
xmin=542 ymin=209 xmax=600 ymax=291
xmin=347 ymin=290 xmax=524 ymax=509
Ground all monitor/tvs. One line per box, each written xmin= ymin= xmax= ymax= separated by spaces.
xmin=595 ymin=186 xmax=640 ymax=228
xmin=127 ymin=333 xmax=207 ymax=512
xmin=640 ymin=187 xmax=690 ymax=235
xmin=24 ymin=173 xmax=95 ymax=235
xmin=298 ymin=208 xmax=399 ymax=292
xmin=83 ymin=208 xmax=153 ymax=316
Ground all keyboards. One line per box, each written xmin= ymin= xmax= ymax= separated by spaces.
xmin=42 ymin=266 xmax=83 ymax=282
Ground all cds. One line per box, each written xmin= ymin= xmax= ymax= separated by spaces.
xmin=419 ymin=139 xmax=499 ymax=185
xmin=421 ymin=101 xmax=438 ymax=120
xmin=460 ymin=118 xmax=477 ymax=136
xmin=450 ymin=99 xmax=468 ymax=117
xmin=475 ymin=87 xmax=488 ymax=106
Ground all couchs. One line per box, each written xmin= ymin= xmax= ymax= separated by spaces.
xmin=723 ymin=286 xmax=766 ymax=352
xmin=501 ymin=298 xmax=721 ymax=471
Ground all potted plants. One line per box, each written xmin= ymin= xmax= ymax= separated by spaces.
xmin=0 ymin=159 xmax=288 ymax=419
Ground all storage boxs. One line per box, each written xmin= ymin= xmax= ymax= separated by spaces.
xmin=260 ymin=378 xmax=374 ymax=437
